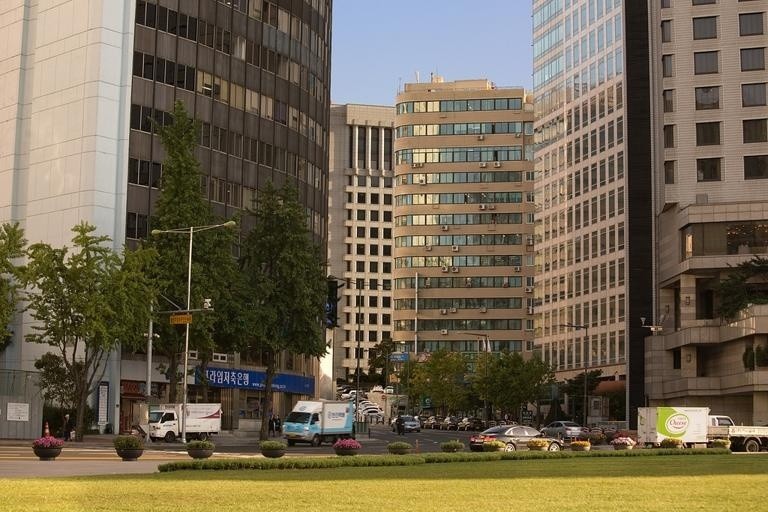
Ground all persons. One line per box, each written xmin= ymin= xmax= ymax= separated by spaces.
xmin=62 ymin=413 xmax=73 ymax=442
xmin=269 ymin=413 xmax=282 ymax=437
xmin=395 ymin=415 xmax=406 ymax=436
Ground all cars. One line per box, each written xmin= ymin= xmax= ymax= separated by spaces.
xmin=392 ymin=413 xmax=590 ymax=452
xmin=336 ymin=382 xmax=395 ymax=424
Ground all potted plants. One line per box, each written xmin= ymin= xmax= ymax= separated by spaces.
xmin=185 ymin=438 xmax=215 ymax=460
xmin=259 ymin=440 xmax=288 ymax=457
xmin=440 ymin=440 xmax=465 ymax=452
xmin=742 ymin=351 xmax=754 ymax=372
xmin=482 ymin=440 xmax=506 ymax=451
xmin=756 ymin=345 xmax=768 ymax=370
xmin=113 ymin=435 xmax=144 ymax=461
xmin=712 ymin=439 xmax=731 ymax=448
xmin=387 ymin=442 xmax=413 ymax=455
xmin=661 ymin=439 xmax=683 ymax=448
xmin=527 ymin=439 xmax=547 ymax=450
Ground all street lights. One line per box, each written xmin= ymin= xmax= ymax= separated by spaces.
xmin=152 ymin=222 xmax=239 ymax=444
xmin=557 ymin=323 xmax=588 ymax=427
xmin=456 ymin=331 xmax=488 ymax=429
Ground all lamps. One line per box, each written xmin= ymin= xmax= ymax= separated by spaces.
xmin=665 ymin=304 xmax=669 ymax=314
xmin=687 ymin=354 xmax=691 ymax=363
xmin=686 ymin=296 xmax=690 ymax=305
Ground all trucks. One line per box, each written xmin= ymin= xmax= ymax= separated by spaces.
xmin=282 ymin=400 xmax=355 ymax=446
xmin=148 ymin=402 xmax=222 ymax=441
xmin=636 ymin=406 xmax=735 ymax=447
xmin=707 ymin=424 xmax=768 ymax=452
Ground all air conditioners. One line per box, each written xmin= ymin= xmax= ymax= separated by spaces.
xmin=420 ymin=133 xmax=533 ymax=335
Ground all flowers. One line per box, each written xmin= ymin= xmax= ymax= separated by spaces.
xmin=609 ymin=437 xmax=637 ymax=448
xmin=32 ymin=436 xmax=64 ymax=448
xmin=332 ymin=437 xmax=362 ymax=449
xmin=570 ymin=441 xmax=591 ymax=449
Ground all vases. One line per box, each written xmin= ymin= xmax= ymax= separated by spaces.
xmin=336 ymin=449 xmax=359 ymax=455
xmin=614 ymin=446 xmax=633 ymax=449
xmin=572 ymin=446 xmax=590 ymax=451
xmin=34 ymin=447 xmax=61 ymax=461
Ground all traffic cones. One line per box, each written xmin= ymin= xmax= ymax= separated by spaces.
xmin=43 ymin=419 xmax=51 ymax=436
